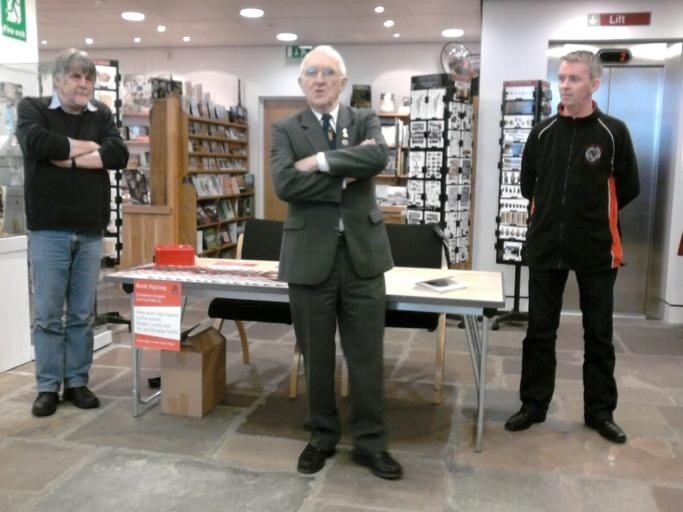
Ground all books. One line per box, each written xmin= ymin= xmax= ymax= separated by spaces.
xmin=188 ymin=102 xmax=249 ymax=259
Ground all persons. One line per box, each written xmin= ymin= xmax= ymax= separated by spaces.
xmin=267 ymin=43 xmax=404 ymax=479
xmin=18 ymin=48 xmax=129 ymax=417
xmin=503 ymin=51 xmax=640 ymax=443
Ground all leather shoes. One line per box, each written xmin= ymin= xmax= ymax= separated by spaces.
xmin=298 ymin=441 xmax=336 ymax=474
xmin=31 ymin=392 xmax=59 ymax=417
xmin=505 ymin=409 xmax=546 ymax=432
xmin=63 ymin=386 xmax=99 ymax=409
xmin=351 ymin=450 xmax=402 ymax=478
xmin=585 ymin=420 xmax=626 ymax=444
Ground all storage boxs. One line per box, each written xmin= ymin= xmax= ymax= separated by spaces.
xmin=161 ymin=323 xmax=226 ymax=419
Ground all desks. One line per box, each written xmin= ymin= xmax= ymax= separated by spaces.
xmin=102 ymin=256 xmax=507 ymax=455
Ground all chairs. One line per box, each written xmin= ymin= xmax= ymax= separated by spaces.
xmin=340 ymin=222 xmax=448 ymax=403
xmin=208 ymin=219 xmax=302 ymax=399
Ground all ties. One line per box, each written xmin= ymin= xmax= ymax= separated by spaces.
xmin=321 ymin=114 xmax=336 ymax=150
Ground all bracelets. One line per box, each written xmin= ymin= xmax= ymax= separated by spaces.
xmin=72 ymin=158 xmax=77 ymax=171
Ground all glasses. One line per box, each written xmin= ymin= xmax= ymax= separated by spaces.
xmin=306 ymin=67 xmax=336 ymax=77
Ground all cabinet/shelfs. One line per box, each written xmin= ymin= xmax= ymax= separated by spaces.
xmin=370 ymin=109 xmax=411 ymax=225
xmin=96 ymin=97 xmax=254 ymax=293
